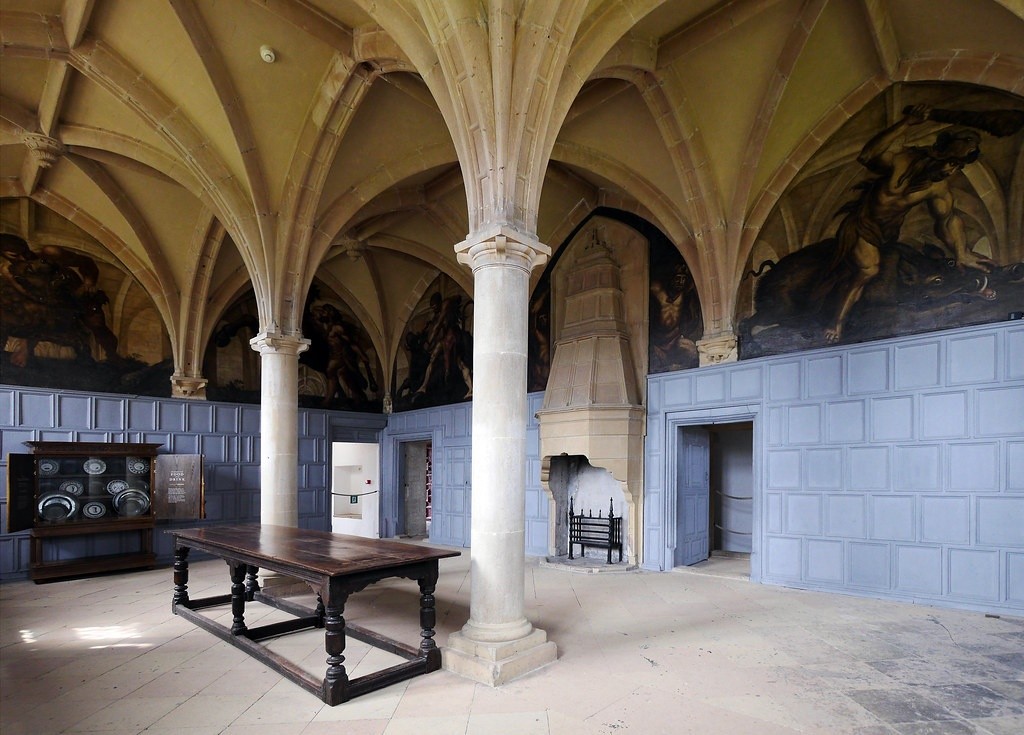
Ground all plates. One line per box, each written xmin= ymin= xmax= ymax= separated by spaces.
xmin=112 ymin=488 xmax=151 ymax=517
xmin=83 ymin=459 xmax=107 ymax=474
xmin=83 ymin=502 xmax=106 ymax=518
xmin=128 ymin=458 xmax=150 ymax=473
xmin=107 ymin=480 xmax=129 ymax=495
xmin=85 ymin=481 xmax=106 ymax=496
xmin=36 ymin=489 xmax=80 ymax=521
xmin=61 ymin=456 xmax=82 ymax=474
xmin=39 ymin=457 xmax=60 ymax=475
xmin=40 ymin=479 xmax=58 ymax=495
xmin=59 ymin=480 xmax=84 ymax=496
xmin=129 ymin=480 xmax=150 ymax=491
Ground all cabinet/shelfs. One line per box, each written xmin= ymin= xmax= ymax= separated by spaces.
xmin=6 ymin=440 xmax=206 ymax=584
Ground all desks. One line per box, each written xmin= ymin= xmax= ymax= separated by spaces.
xmin=164 ymin=524 xmax=462 ymax=708
